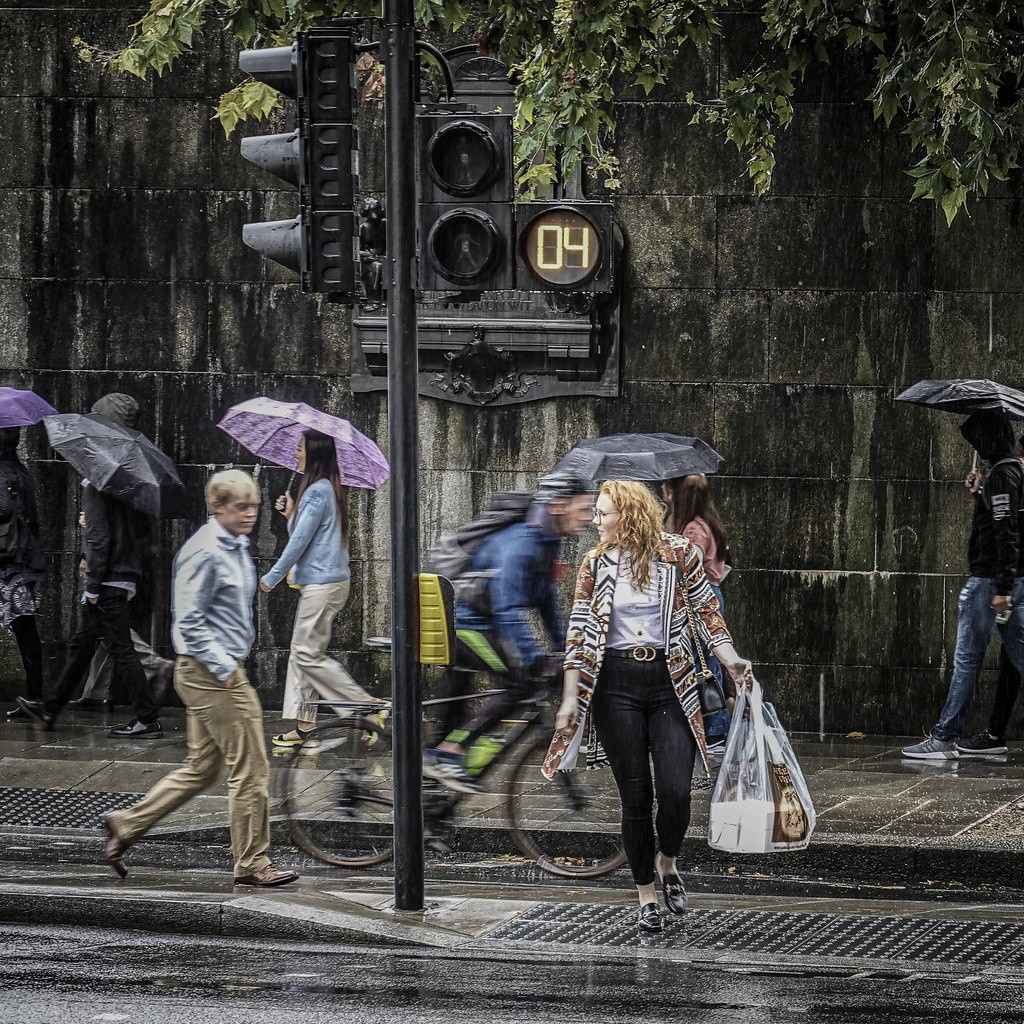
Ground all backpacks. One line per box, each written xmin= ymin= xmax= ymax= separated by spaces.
xmin=432 ymin=489 xmax=535 ymax=619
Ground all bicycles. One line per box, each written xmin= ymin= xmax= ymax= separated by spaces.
xmin=270 ymin=635 xmax=658 ymax=881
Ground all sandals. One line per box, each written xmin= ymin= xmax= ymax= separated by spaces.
xmin=364 ymin=697 xmax=392 ymax=746
xmin=271 ymin=725 xmax=320 ymax=748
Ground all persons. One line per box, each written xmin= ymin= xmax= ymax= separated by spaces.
xmin=99 ymin=470 xmax=299 ymax=886
xmin=259 ymin=429 xmax=391 ymax=747
xmin=16 ymin=480 xmax=164 ymax=739
xmin=662 ymin=473 xmax=730 ymax=748
xmin=900 ymin=408 xmax=1024 ymax=759
xmin=421 ymin=472 xmax=593 ymax=853
xmin=66 ymin=389 xmax=174 ymax=714
xmin=955 ymin=436 xmax=1023 ymax=755
xmin=540 ymin=479 xmax=756 ymax=931
xmin=0 ymin=425 xmax=42 ymax=717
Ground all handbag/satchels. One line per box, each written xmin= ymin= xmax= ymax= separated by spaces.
xmin=695 ymin=670 xmax=731 ymax=746
xmin=707 ymin=674 xmax=816 ymax=854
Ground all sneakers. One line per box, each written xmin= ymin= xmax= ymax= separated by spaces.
xmin=956 ymin=728 xmax=1009 ymax=754
xmin=901 ymin=725 xmax=960 ymax=760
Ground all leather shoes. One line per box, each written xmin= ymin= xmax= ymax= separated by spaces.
xmin=152 ymin=660 xmax=175 ymax=707
xmin=64 ymin=697 xmax=115 ymax=713
xmin=108 ymin=719 xmax=163 ymax=739
xmin=655 ymin=864 xmax=689 ymax=916
xmin=102 ymin=813 xmax=129 ymax=879
xmin=233 ymin=865 xmax=299 ymax=888
xmin=6 ymin=707 xmax=33 ymax=719
xmin=16 ymin=696 xmax=56 ymax=730
xmin=637 ymin=900 xmax=665 ymax=933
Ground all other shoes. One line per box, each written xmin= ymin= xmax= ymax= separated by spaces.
xmin=420 ymin=741 xmax=488 ymax=796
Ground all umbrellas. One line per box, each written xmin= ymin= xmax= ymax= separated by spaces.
xmin=42 ymin=413 xmax=192 ymax=521
xmin=894 ymin=379 xmax=1023 ymax=486
xmin=0 ymin=386 xmax=57 ymax=428
xmin=538 ymin=434 xmax=724 ymax=481
xmin=216 ymin=395 xmax=390 ymax=513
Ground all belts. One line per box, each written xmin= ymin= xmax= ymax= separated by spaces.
xmin=604 ymin=646 xmax=666 ymax=663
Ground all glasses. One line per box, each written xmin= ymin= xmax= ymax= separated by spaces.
xmin=591 ymin=506 xmax=618 ymax=526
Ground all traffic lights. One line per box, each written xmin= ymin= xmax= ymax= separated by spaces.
xmin=411 ymin=106 xmax=615 ymax=299
xmin=237 ymin=25 xmax=361 ymax=302
